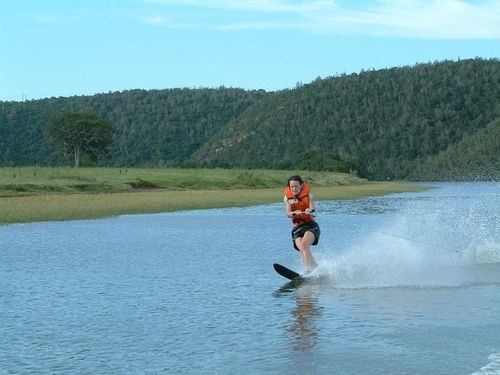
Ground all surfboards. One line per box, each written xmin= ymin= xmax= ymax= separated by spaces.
xmin=274 ymin=263 xmax=299 ymax=282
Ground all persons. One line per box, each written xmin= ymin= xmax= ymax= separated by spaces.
xmin=284 ymin=176 xmax=321 ymax=272
